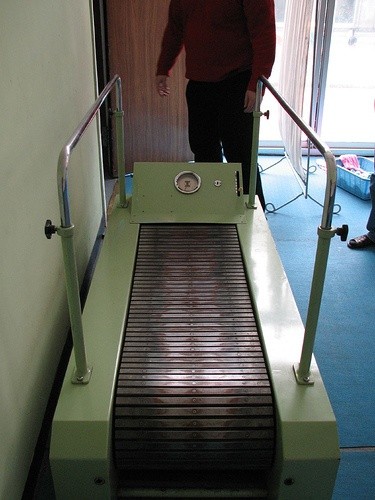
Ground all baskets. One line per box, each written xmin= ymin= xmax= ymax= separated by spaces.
xmin=334 ymin=156 xmax=374 ymax=201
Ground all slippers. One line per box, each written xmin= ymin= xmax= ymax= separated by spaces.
xmin=347 ymin=234 xmax=375 ymax=249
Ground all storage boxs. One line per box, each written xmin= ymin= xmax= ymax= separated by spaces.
xmin=333 ymin=155 xmax=375 ymax=201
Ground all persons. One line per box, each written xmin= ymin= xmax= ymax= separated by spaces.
xmin=346 ymin=167 xmax=375 ymax=250
xmin=154 ymin=0 xmax=276 ymax=212
